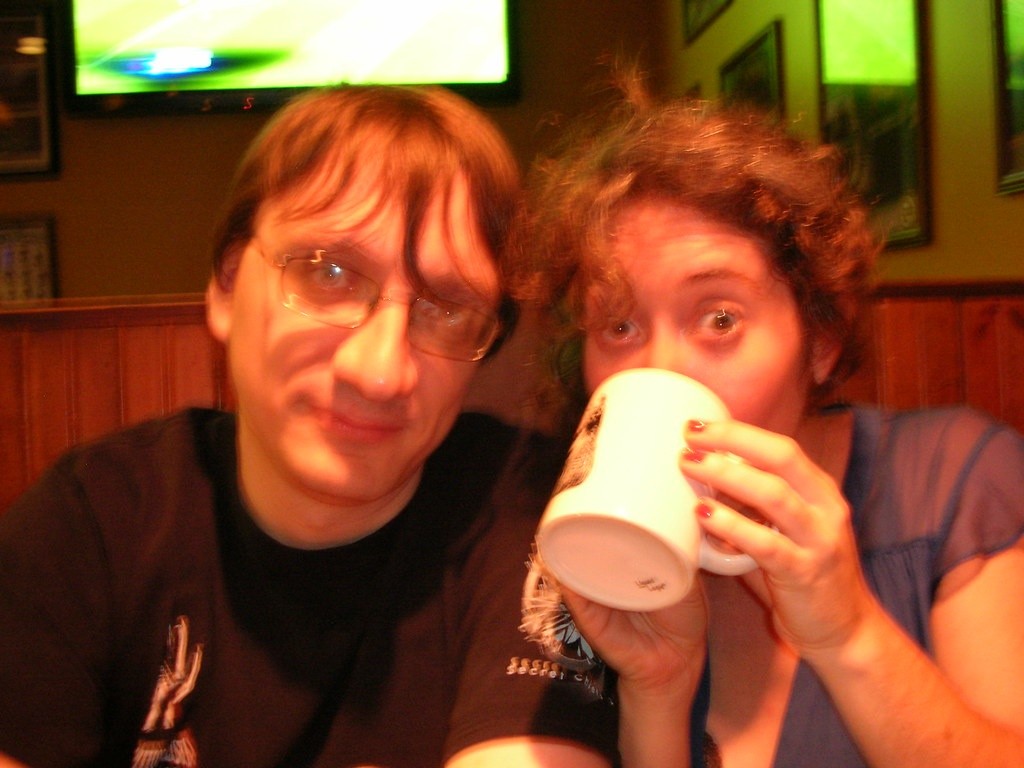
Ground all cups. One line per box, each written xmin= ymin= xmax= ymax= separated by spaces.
xmin=535 ymin=368 xmax=782 ymax=615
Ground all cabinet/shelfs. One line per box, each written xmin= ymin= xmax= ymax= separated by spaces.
xmin=0 ymin=276 xmax=1024 ymax=518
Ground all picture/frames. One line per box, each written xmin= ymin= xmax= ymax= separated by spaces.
xmin=716 ymin=21 xmax=784 ymax=123
xmin=0 ymin=12 xmax=62 ymax=182
xmin=680 ymin=0 xmax=734 ymax=46
xmin=989 ymin=0 xmax=1024 ymax=198
xmin=814 ymin=0 xmax=933 ymax=250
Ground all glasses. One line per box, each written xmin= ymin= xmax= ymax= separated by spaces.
xmin=249 ymin=237 xmax=503 ymax=362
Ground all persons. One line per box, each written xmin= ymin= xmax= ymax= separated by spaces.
xmin=502 ymin=101 xmax=1024 ymax=768
xmin=0 ymin=88 xmax=621 ymax=768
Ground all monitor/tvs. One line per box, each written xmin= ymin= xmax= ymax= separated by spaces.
xmin=42 ymin=1 xmax=522 ymax=118
